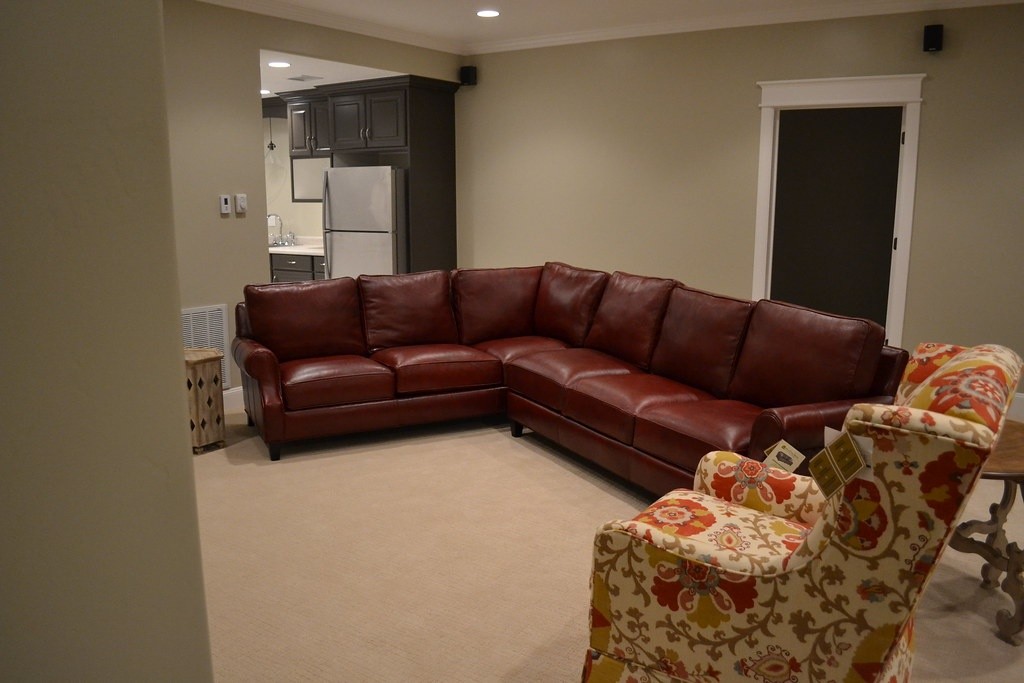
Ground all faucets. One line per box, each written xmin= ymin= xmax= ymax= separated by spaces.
xmin=267 ymin=213 xmax=282 ymax=245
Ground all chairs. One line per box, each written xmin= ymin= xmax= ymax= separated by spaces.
xmin=578 ymin=341 xmax=1024 ymax=683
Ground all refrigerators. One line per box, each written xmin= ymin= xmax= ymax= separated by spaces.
xmin=322 ymin=166 xmax=396 ymax=279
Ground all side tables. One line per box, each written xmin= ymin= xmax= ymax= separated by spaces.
xmin=946 ymin=418 xmax=1024 ymax=646
xmin=186 ymin=346 xmax=225 ymax=455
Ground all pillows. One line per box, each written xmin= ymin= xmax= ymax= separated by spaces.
xmin=583 ymin=270 xmax=684 ymax=370
xmin=534 ymin=262 xmax=611 ymax=346
xmin=730 ymin=297 xmax=886 ymax=406
xmin=242 ymin=275 xmax=367 ymax=362
xmin=650 ymin=284 xmax=754 ymax=397
xmin=450 ymin=266 xmax=544 ymax=345
xmin=357 ymin=269 xmax=460 ymax=352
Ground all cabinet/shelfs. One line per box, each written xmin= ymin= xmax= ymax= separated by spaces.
xmin=287 ymin=98 xmax=330 ymax=159
xmin=327 ymin=87 xmax=409 ymax=151
xmin=271 ymin=254 xmax=313 ymax=282
xmin=313 ymin=256 xmax=325 ymax=280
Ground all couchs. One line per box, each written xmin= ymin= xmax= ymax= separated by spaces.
xmin=230 ymin=302 xmax=909 ymax=498
